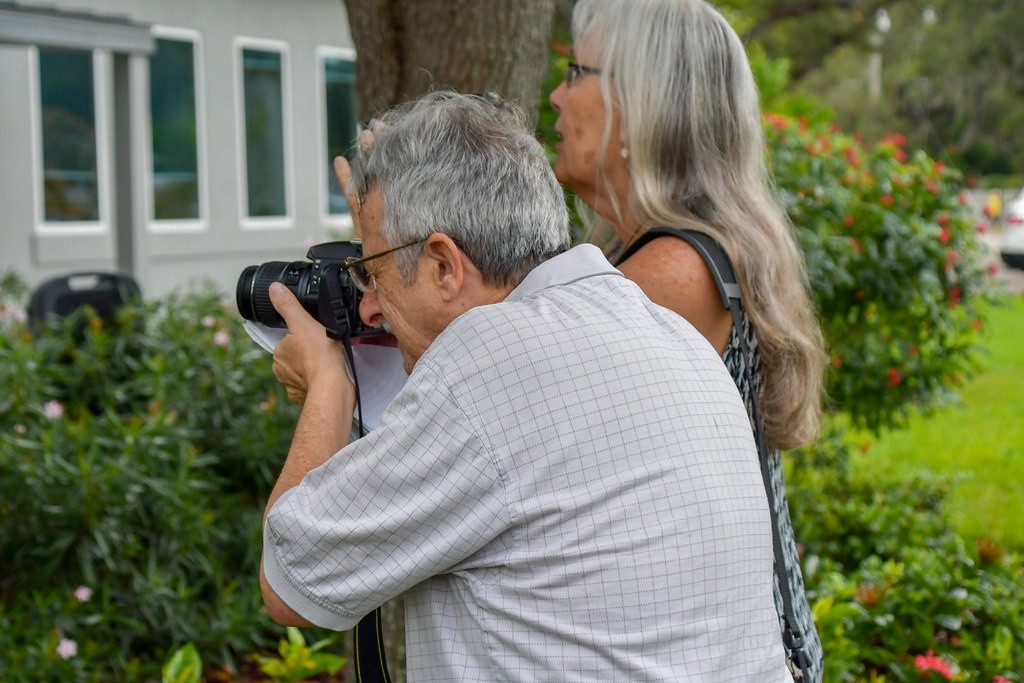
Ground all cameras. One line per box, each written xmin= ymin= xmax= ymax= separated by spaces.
xmin=236 ymin=241 xmax=389 ymax=344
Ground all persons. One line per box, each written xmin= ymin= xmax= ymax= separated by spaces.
xmin=548 ymin=1 xmax=830 ymax=682
xmin=258 ymin=88 xmax=799 ymax=683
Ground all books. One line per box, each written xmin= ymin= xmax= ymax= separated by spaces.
xmin=243 ymin=321 xmax=410 ymax=442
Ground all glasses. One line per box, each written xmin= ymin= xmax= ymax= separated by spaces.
xmin=343 ymin=237 xmax=425 ymax=294
xmin=566 ymin=61 xmax=614 ymax=88
